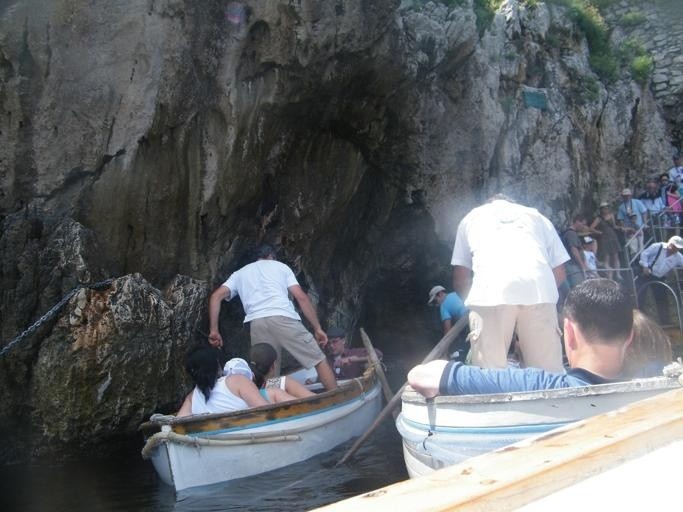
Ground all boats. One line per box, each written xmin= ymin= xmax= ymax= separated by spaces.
xmin=140 ymin=347 xmax=385 ymax=494
xmin=393 ymin=366 xmax=682 ymax=483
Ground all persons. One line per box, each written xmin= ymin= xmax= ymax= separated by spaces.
xmin=618 ymin=309 xmax=672 ymax=379
xmin=175 ymin=347 xmax=267 ymax=418
xmin=247 ymin=343 xmax=317 ymax=399
xmin=425 ymin=285 xmax=470 ymax=363
xmin=326 ymin=329 xmax=382 ymax=382
xmin=561 ymin=151 xmax=682 ymax=327
xmin=406 ymin=279 xmax=633 ymax=395
xmin=222 ymin=358 xmax=299 ymax=405
xmin=449 ymin=191 xmax=571 ymax=372
xmin=205 ymin=244 xmax=337 ymax=395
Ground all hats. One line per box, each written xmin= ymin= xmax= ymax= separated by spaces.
xmin=668 ymin=235 xmax=683 ymax=249
xmin=222 ymin=357 xmax=253 ymax=382
xmin=599 ymin=202 xmax=610 ymax=208
xmin=621 ymin=188 xmax=632 ymax=196
xmin=427 ymin=286 xmax=446 ymax=307
xmin=325 ymin=327 xmax=346 ymax=338
xmin=582 ymin=236 xmax=593 ymax=244
